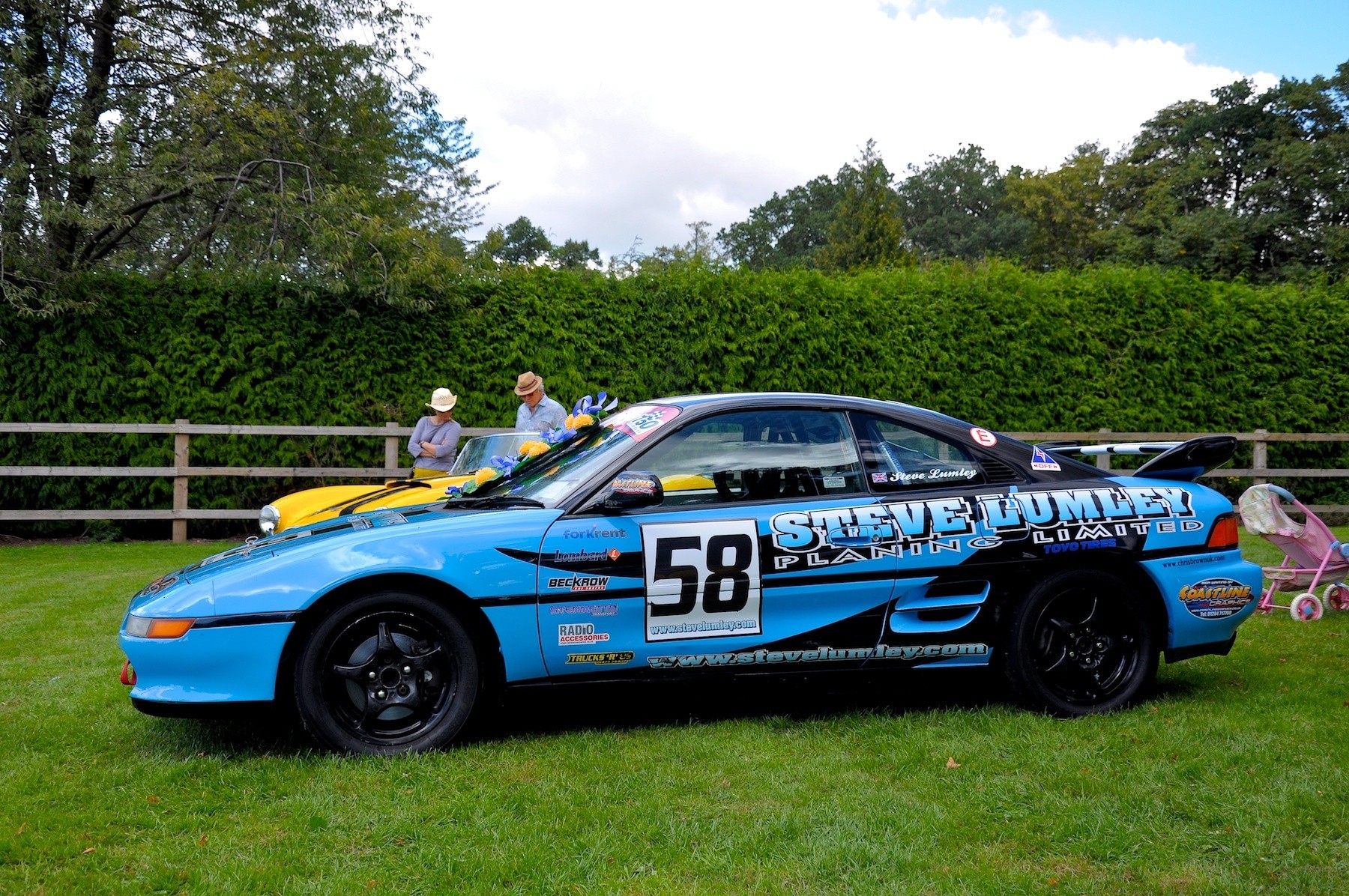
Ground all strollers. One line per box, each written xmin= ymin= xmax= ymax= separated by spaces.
xmin=1241 ymin=483 xmax=1349 ymax=623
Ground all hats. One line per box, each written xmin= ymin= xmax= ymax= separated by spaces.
xmin=425 ymin=387 xmax=457 ymax=412
xmin=514 ymin=371 xmax=543 ymax=396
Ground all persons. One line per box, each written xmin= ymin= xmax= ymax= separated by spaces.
xmin=514 ymin=371 xmax=567 ymax=456
xmin=407 ymin=388 xmax=462 ymax=477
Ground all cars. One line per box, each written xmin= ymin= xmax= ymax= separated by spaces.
xmin=118 ymin=392 xmax=1263 ymax=760
xmin=259 ymin=433 xmax=713 ymax=539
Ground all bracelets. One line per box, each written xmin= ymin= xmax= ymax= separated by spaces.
xmin=420 ymin=440 xmax=425 ymax=448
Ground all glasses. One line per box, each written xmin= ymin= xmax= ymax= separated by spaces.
xmin=521 ymin=392 xmax=534 ymax=397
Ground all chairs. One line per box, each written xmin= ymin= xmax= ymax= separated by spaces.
xmin=782 ymin=441 xmax=848 ymax=499
xmin=711 ymin=441 xmax=781 ymax=502
